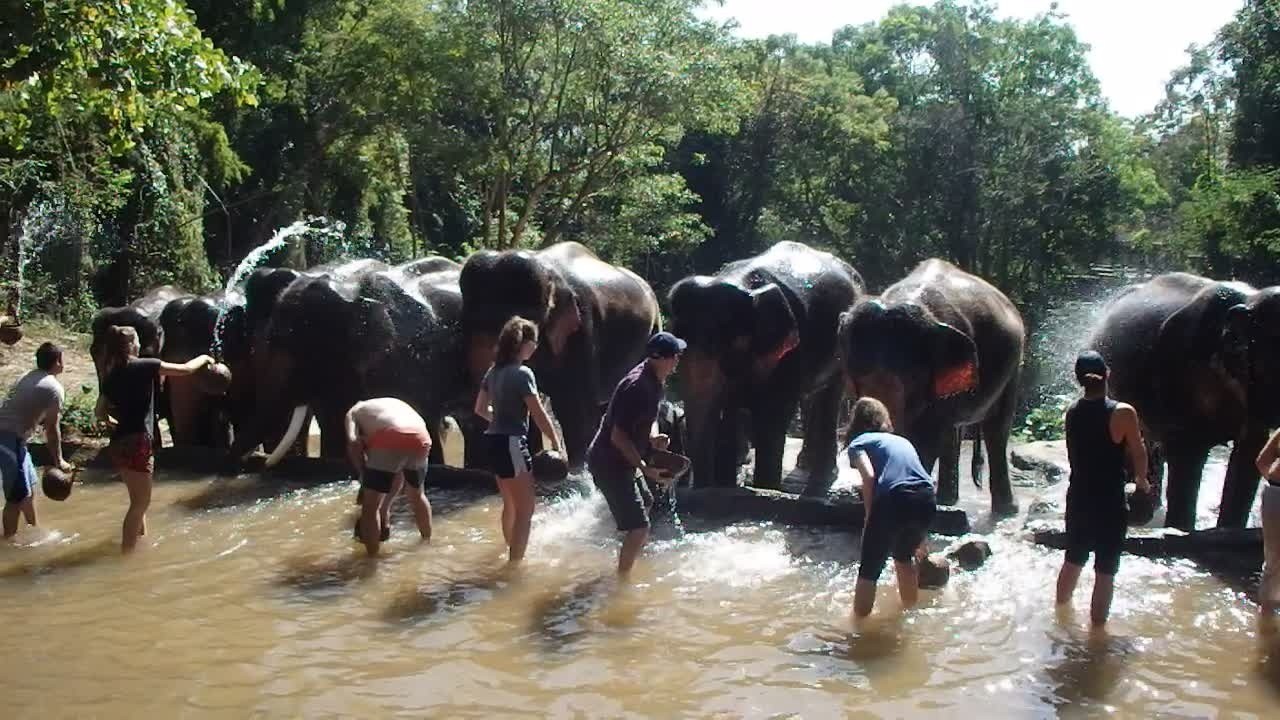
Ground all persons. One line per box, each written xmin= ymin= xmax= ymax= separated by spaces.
xmin=102 ymin=325 xmax=215 ymax=544
xmin=586 ymin=332 xmax=687 ymax=569
xmin=1254 ymin=428 xmax=1280 ymax=600
xmin=475 ymin=316 xmax=559 ymax=562
xmin=0 ymin=342 xmax=65 ymax=538
xmin=1056 ymin=350 xmax=1152 ymax=626
xmin=843 ymin=395 xmax=937 ymax=617
xmin=345 ymin=397 xmax=433 ymax=552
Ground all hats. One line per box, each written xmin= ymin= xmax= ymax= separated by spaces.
xmin=1075 ymin=351 xmax=1107 ymax=378
xmin=648 ymin=332 xmax=687 ymax=353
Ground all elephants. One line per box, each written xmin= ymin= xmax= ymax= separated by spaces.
xmin=89 ymin=241 xmax=1279 ymax=529
